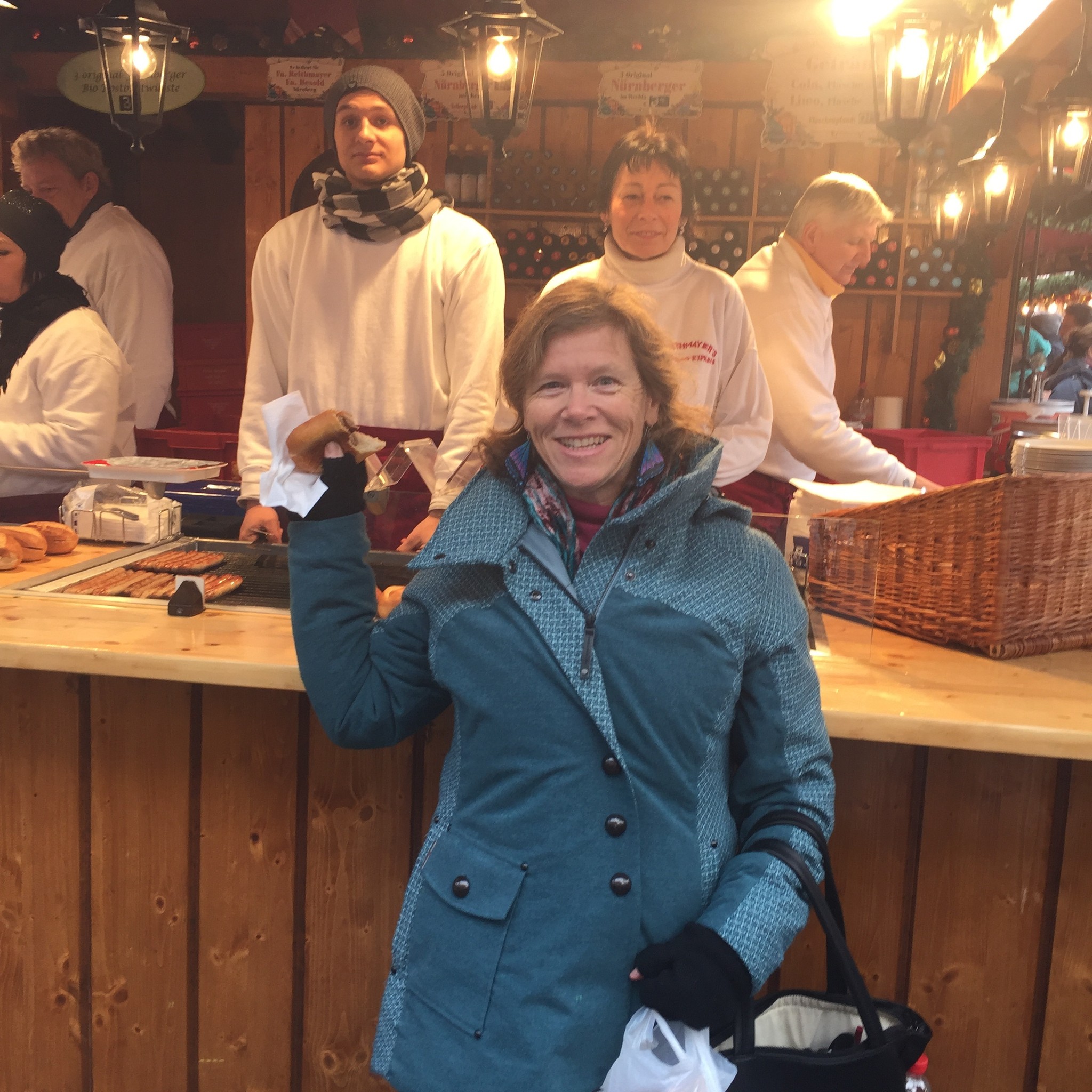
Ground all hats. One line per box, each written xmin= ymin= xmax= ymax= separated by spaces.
xmin=321 ymin=64 xmax=427 ymax=164
xmin=0 ymin=188 xmax=68 ymax=271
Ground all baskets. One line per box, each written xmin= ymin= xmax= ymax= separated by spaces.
xmin=807 ymin=471 xmax=1092 ymax=659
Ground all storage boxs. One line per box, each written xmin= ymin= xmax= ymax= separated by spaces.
xmin=855 ymin=422 xmax=993 ymax=486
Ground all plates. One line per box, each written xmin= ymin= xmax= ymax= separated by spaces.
xmin=1010 ymin=438 xmax=1092 ymax=475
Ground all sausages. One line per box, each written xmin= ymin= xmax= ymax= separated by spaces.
xmin=57 ymin=540 xmax=244 ymax=612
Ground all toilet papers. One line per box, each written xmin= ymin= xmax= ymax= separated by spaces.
xmin=869 ymin=394 xmax=910 ymax=438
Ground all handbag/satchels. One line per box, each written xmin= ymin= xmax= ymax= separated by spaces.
xmin=709 ymin=809 xmax=935 ymax=1092
xmin=599 ymin=1004 xmax=738 ymax=1092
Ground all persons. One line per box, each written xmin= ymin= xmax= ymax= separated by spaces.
xmin=279 ymin=273 xmax=843 ymax=1092
xmin=0 ymin=184 xmax=151 ymax=519
xmin=1005 ymin=294 xmax=1092 ymax=421
xmin=704 ymin=168 xmax=950 ymax=537
xmin=8 ymin=125 xmax=185 ymax=440
xmin=233 ymin=64 xmax=513 ymax=561
xmin=537 ymin=120 xmax=775 ymax=507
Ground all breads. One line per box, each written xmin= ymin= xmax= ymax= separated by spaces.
xmin=0 ymin=513 xmax=78 ymax=572
xmin=373 ymin=580 xmax=411 ymax=617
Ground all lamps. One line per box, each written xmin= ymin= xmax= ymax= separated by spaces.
xmin=77 ymin=0 xmax=190 ymax=155
xmin=439 ymin=1 xmax=564 ymax=163
xmin=868 ymin=2 xmax=986 ymax=163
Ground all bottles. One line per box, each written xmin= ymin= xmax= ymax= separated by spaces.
xmin=442 ymin=143 xmax=970 ymax=294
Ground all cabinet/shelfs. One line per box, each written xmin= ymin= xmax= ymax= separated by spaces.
xmin=427 ymin=149 xmax=973 ymax=354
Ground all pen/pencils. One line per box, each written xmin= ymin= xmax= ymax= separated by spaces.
xmin=854 ymin=1026 xmax=863 ymax=1046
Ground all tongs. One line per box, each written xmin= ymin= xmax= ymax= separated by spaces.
xmin=245 ymin=526 xmax=274 ymax=551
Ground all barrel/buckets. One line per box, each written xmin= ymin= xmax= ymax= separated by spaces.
xmin=986 ymin=398 xmax=1075 ymax=475
xmin=1004 ymin=418 xmax=1060 ymax=475
